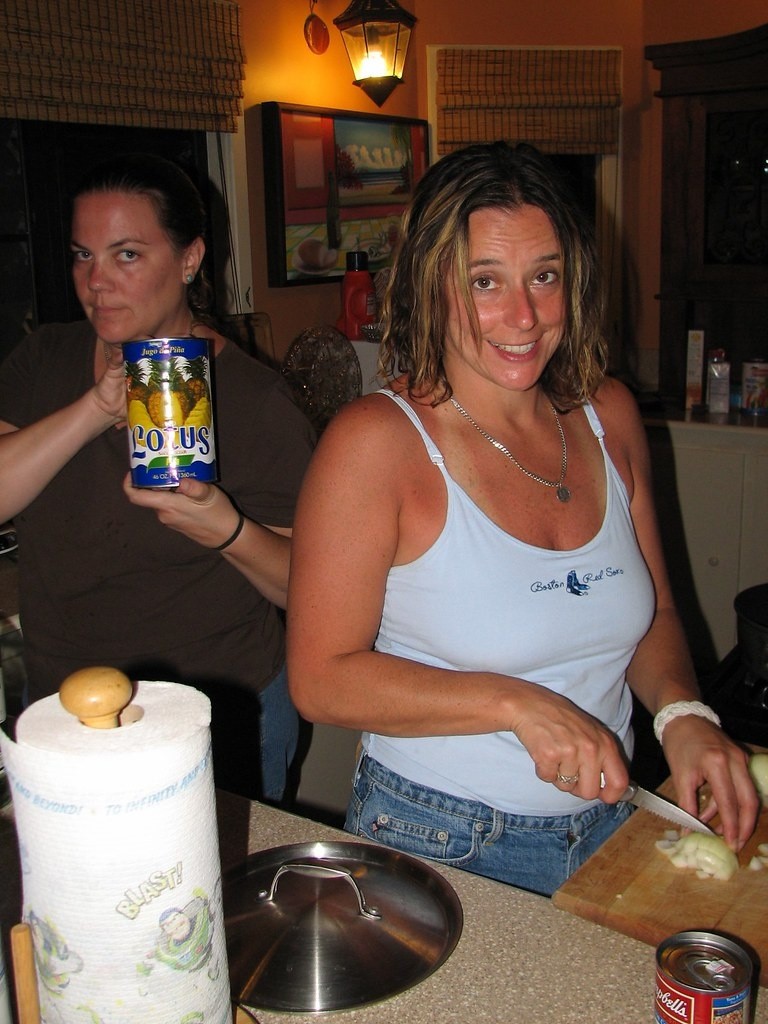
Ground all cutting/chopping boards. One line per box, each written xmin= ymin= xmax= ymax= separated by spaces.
xmin=550 ymin=740 xmax=768 ymax=987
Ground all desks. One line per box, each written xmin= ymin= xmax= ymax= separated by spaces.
xmin=0 ymin=792 xmax=768 ymax=1024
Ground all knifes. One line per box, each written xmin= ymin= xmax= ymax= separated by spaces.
xmin=595 ymin=771 xmax=717 ymax=836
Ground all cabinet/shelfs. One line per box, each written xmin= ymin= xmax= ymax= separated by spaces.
xmin=637 ymin=436 xmax=768 ymax=701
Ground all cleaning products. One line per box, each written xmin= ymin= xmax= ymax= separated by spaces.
xmin=335 ymin=250 xmax=381 ymax=342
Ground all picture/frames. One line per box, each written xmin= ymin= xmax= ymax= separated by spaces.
xmin=261 ymin=100 xmax=430 ymax=290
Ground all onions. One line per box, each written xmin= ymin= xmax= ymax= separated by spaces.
xmin=656 ymin=829 xmax=768 ymax=881
xmin=747 ymin=752 xmax=768 ymax=803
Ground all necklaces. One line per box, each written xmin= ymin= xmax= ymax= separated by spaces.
xmin=103 ymin=318 xmax=197 ymax=365
xmin=437 ymin=384 xmax=576 ymax=502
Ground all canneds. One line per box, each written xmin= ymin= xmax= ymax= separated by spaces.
xmin=654 ymin=931 xmax=753 ymax=1024
xmin=121 ymin=336 xmax=218 ymax=489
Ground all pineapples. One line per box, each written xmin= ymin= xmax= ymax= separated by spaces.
xmin=124 ymin=356 xmax=211 ymax=446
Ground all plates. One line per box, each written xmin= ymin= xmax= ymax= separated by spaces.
xmin=290 ymin=248 xmax=338 ymax=274
xmin=352 ymin=238 xmax=390 ymax=262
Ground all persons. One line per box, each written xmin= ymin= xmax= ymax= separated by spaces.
xmin=282 ymin=138 xmax=762 ymax=898
xmin=0 ymin=152 xmax=320 ymax=807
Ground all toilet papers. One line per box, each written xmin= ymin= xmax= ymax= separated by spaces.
xmin=0 ymin=676 xmax=237 ymax=1024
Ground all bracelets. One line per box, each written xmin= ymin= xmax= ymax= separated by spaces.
xmin=652 ymin=699 xmax=721 ymax=747
xmin=210 ymin=512 xmax=244 ymax=551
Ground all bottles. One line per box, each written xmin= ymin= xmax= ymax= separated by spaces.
xmin=341 ymin=252 xmax=376 ymax=340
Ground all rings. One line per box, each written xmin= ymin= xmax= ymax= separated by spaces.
xmin=557 ymin=771 xmax=579 ymax=784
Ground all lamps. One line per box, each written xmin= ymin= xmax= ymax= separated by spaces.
xmin=332 ymin=0 xmax=418 ymax=108
